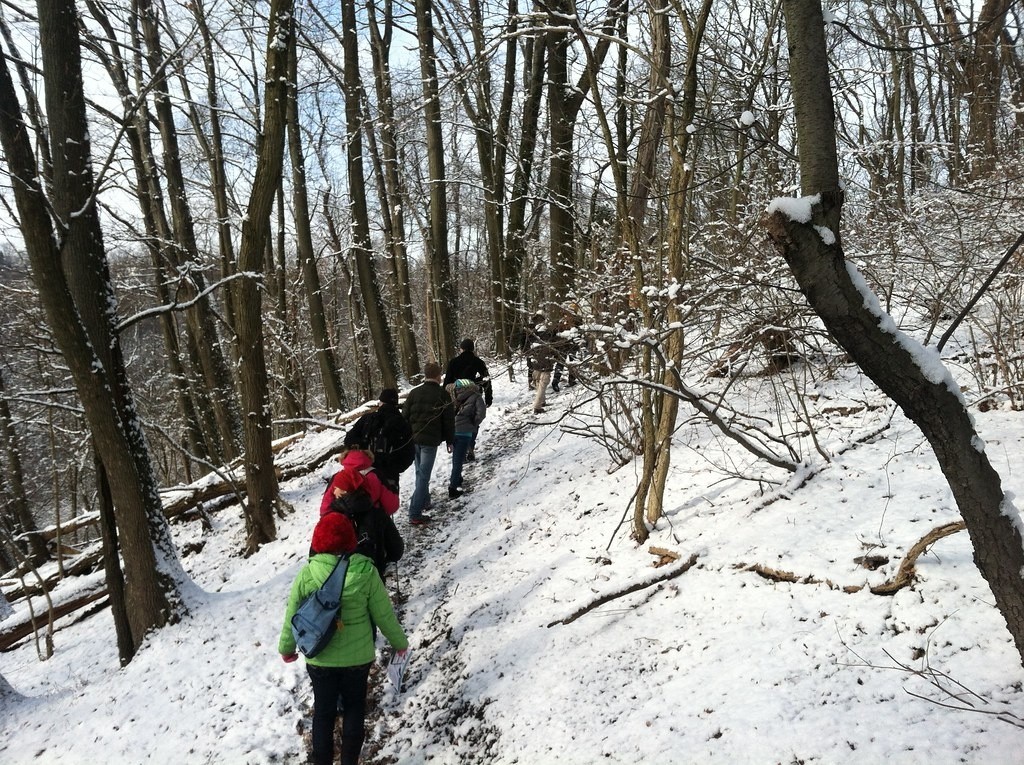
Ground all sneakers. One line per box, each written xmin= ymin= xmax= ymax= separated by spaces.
xmin=466 ymin=452 xmax=476 ymax=461
xmin=409 ymin=515 xmax=431 ymax=524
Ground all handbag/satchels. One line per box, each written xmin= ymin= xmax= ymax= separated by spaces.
xmin=290 ymin=555 xmax=350 ymax=658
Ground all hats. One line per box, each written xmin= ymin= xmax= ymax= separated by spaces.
xmin=379 ymin=388 xmax=399 ymax=405
xmin=460 ymin=338 xmax=474 ymax=352
xmin=568 ymin=304 xmax=579 ymax=312
xmin=311 ymin=512 xmax=357 ymax=554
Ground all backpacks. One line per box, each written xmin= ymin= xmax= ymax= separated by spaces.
xmin=362 ymin=408 xmax=406 ymax=462
xmin=333 ymin=486 xmax=385 ymax=563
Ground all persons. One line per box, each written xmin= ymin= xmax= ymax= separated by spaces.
xmin=276 ymin=511 xmax=409 ymax=765
xmin=443 ymin=338 xmax=494 ymax=463
xmin=343 ymin=387 xmax=416 ymax=496
xmin=519 ymin=302 xmax=588 ymax=414
xmin=308 ymin=468 xmax=405 ymax=718
xmin=320 ymin=430 xmax=402 ymax=518
xmin=444 ymin=364 xmax=487 ymax=501
xmin=402 ymin=362 xmax=456 ymax=526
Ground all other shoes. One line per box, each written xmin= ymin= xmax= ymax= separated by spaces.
xmin=569 ymin=381 xmax=577 ymax=386
xmin=449 ymin=490 xmax=464 ymax=501
xmin=552 ymin=381 xmax=560 ymax=392
xmin=534 ymin=409 xmax=546 ymax=414
xmin=457 ymin=477 xmax=464 ymax=487
